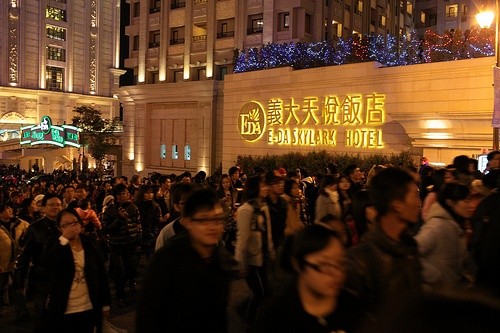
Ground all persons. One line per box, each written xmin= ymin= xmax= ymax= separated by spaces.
xmin=0 ymin=149 xmax=500 ymax=333
xmin=235 ymin=177 xmax=275 ymax=333
xmin=411 ymin=180 xmax=476 ymax=292
xmin=256 ymin=225 xmax=360 ymax=333
xmin=34 ymin=209 xmax=112 ymax=333
xmin=134 ymin=188 xmax=235 ymax=333
xmin=105 ymin=184 xmax=142 ymax=310
xmin=343 ymin=167 xmax=427 ymax=333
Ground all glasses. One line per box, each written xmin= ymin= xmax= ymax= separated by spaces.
xmin=190 ymin=216 xmax=224 ymax=225
xmin=303 ymin=259 xmax=345 ymax=275
xmin=61 ymin=221 xmax=78 ymax=228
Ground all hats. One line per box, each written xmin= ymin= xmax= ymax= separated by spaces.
xmin=279 ymin=168 xmax=286 ymax=175
xmin=34 ymin=194 xmax=45 ymax=204
xmin=103 ymin=195 xmax=114 ymax=206
